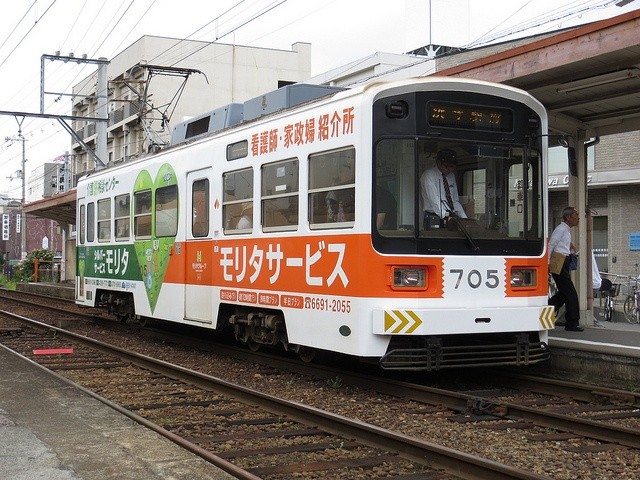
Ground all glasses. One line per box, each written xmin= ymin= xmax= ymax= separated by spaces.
xmin=441 ymin=162 xmax=456 ymax=168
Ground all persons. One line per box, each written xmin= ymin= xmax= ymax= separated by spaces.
xmin=419 ymin=148 xmax=467 ymax=231
xmin=549 ymin=208 xmax=583 ymax=331
xmin=235 ymin=201 xmax=252 ymax=229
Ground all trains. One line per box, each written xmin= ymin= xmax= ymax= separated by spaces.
xmin=74 ymin=77 xmax=555 ymax=373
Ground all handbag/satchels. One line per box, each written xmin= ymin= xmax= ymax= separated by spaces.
xmin=570 ymin=253 xmax=577 ymax=270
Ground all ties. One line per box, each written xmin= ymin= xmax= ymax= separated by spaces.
xmin=442 ymin=171 xmax=454 ymax=210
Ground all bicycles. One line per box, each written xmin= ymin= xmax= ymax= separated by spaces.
xmin=617 ymin=275 xmax=640 ymax=324
xmin=599 ymin=272 xmax=622 ymax=321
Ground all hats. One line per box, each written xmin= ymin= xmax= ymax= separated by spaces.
xmin=240 ymin=202 xmax=252 ymax=217
xmin=436 ymin=148 xmax=457 ymax=166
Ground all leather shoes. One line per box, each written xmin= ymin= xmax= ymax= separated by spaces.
xmin=565 ymin=324 xmax=584 ymax=331
